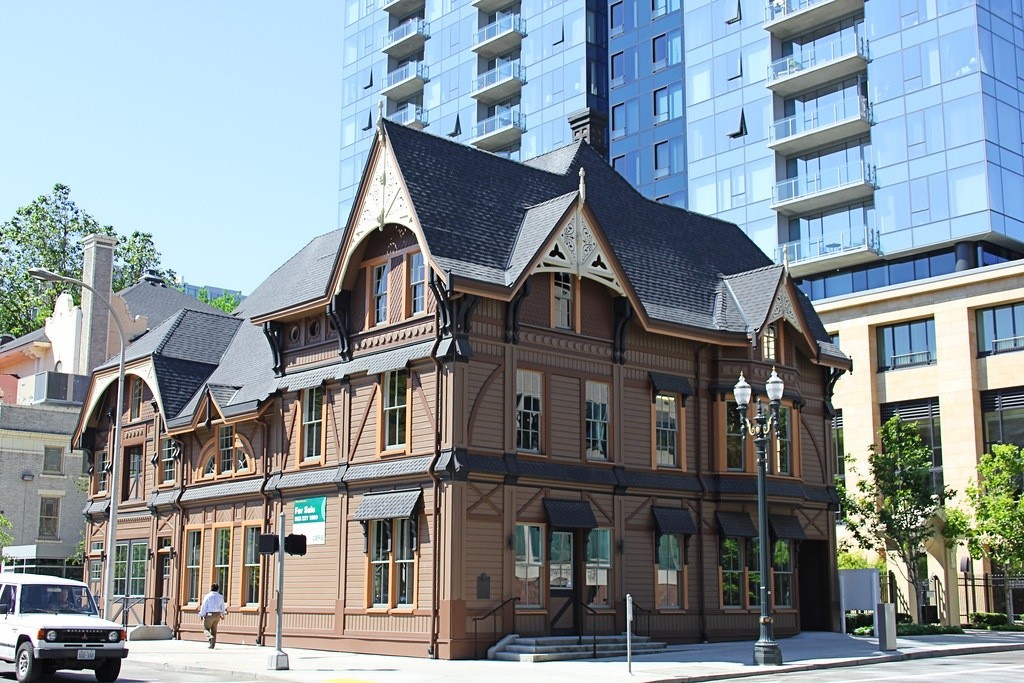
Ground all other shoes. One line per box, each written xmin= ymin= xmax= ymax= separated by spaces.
xmin=208 ymin=646 xmax=214 ymax=649
xmin=210 ymin=636 xmax=215 ymax=646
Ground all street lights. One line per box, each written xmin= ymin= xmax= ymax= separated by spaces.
xmin=27 ymin=265 xmax=126 ymax=621
xmin=732 ymin=366 xmax=785 ymax=666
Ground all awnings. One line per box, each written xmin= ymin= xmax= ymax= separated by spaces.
xmin=543 ymin=497 xmax=599 ymax=528
xmin=653 ymin=505 xmax=698 ymax=537
xmin=354 ymin=490 xmax=422 ymax=525
xmin=714 ymin=511 xmax=808 ymax=539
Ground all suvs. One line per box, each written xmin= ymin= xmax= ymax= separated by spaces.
xmin=0 ymin=571 xmax=129 ymax=683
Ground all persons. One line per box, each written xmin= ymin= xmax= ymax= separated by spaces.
xmin=199 ymin=584 xmax=225 ymax=649
xmin=48 ymin=590 xmax=74 ymax=611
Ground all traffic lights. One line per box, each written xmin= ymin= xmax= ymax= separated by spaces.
xmin=285 ymin=533 xmax=308 ymax=556
xmin=258 ymin=532 xmax=279 ymax=555
